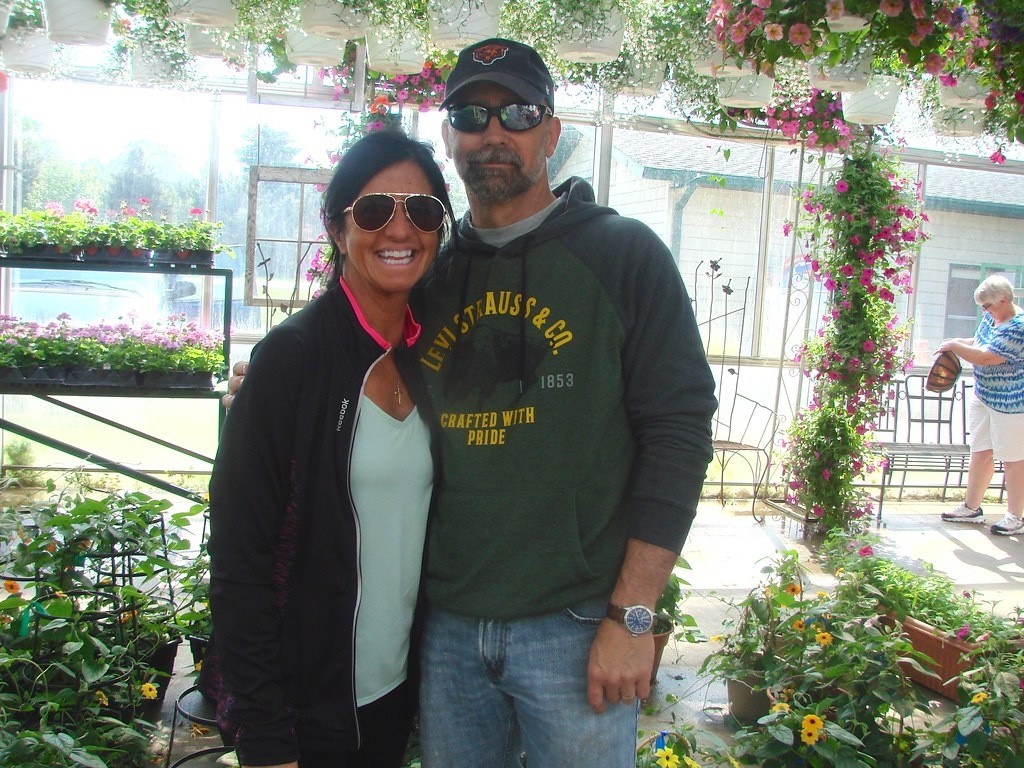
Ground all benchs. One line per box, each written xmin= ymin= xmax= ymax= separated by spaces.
xmin=850 ymin=374 xmax=1007 ymax=528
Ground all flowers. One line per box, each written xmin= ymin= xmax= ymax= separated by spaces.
xmin=0 ymin=1 xmax=1024 ymax=768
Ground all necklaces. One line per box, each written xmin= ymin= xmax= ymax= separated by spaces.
xmin=379 ymin=360 xmax=401 ymax=405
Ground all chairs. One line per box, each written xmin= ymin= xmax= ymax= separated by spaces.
xmin=689 ymin=257 xmax=780 ymax=522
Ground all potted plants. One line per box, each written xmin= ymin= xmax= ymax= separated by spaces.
xmin=649 ymin=555 xmax=692 ymax=682
xmin=876 ymin=561 xmax=1024 ymax=705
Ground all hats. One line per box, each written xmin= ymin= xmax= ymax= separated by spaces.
xmin=437 ymin=37 xmax=554 ymax=116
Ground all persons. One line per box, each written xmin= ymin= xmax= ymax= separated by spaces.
xmin=221 ymin=38 xmax=718 ymax=768
xmin=195 ymin=132 xmax=459 ymax=768
xmin=933 ymin=275 xmax=1024 ymax=536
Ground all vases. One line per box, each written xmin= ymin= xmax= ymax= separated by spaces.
xmin=808 ymin=49 xmax=874 ymax=92
xmin=185 ymin=633 xmax=209 ymax=698
xmin=285 ymin=25 xmax=346 ymax=66
xmin=812 ymin=9 xmax=875 ymax=32
xmin=300 ymin=0 xmax=371 ymax=39
xmin=170 ymin=1 xmax=238 ymax=27
xmin=42 ymin=0 xmax=113 ymax=44
xmin=555 ymin=0 xmax=625 ymax=63
xmin=717 ymin=69 xmax=774 ymax=108
xmin=631 ymin=58 xmax=666 ymax=85
xmin=183 ymin=24 xmax=245 ymax=57
xmin=693 ymin=51 xmax=754 ymax=78
xmin=615 ymin=82 xmax=661 ymax=96
xmin=140 ymin=636 xmax=182 ymax=704
xmin=427 ymin=0 xmax=502 ymax=51
xmin=0 ymin=27 xmax=54 ymax=73
xmin=365 ymin=18 xmax=426 ymax=75
xmin=760 ymin=619 xmax=802 ymax=659
xmin=841 ymin=75 xmax=908 ymax=125
xmin=938 ymin=75 xmax=993 ymax=109
xmin=932 ymin=111 xmax=985 ymax=136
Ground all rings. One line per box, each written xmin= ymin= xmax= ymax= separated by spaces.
xmin=622 ymin=689 xmax=635 ymax=705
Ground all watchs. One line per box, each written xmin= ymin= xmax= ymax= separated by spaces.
xmin=606 ymin=603 xmax=659 ymax=638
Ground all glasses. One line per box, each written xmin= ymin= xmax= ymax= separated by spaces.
xmin=447 ymin=104 xmax=551 ymax=132
xmin=982 ymin=303 xmax=993 ymax=311
xmin=340 ymin=193 xmax=448 ymax=233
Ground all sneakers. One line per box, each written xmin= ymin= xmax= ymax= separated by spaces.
xmin=940 ymin=503 xmax=986 ymax=523
xmin=990 ymin=511 xmax=1024 ymax=536
